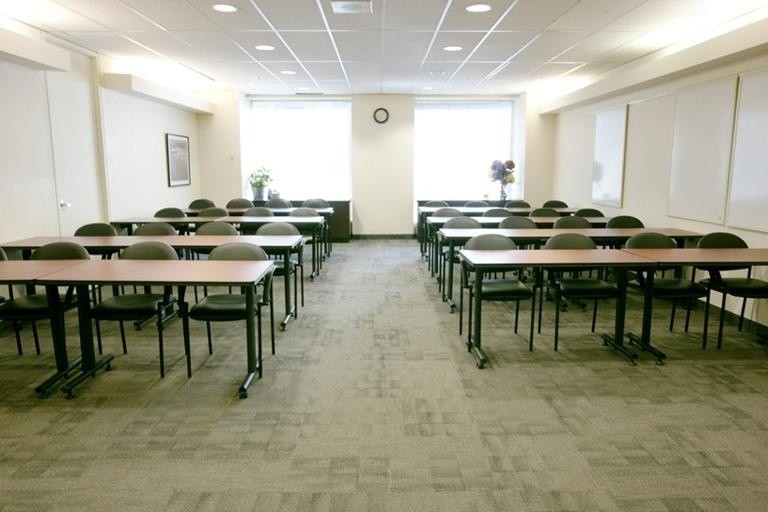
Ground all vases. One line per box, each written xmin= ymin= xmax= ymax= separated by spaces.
xmin=500 ymin=190 xmax=506 ymax=200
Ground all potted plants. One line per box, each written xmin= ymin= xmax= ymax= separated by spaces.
xmin=249 ymin=168 xmax=273 ymax=201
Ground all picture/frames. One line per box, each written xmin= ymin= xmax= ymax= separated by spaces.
xmin=166 ymin=134 xmax=191 ymax=187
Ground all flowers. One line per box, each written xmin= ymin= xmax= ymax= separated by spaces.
xmin=489 ymin=160 xmax=515 ymax=190
